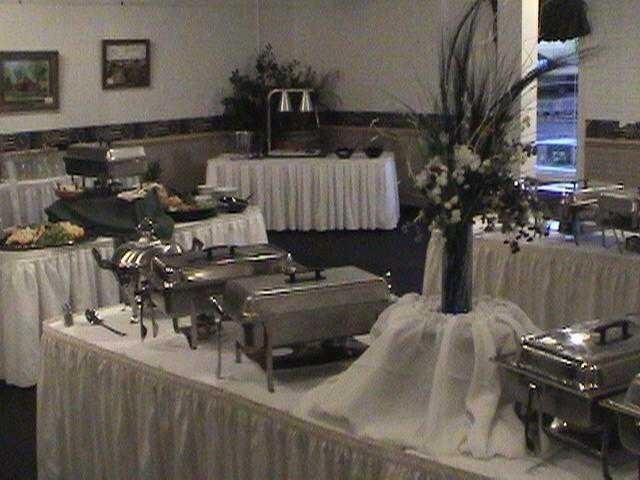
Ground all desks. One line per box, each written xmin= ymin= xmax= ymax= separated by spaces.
xmin=3 ymin=173 xmax=141 ymax=228
xmin=205 ymin=153 xmax=403 ymax=234
xmin=34 ymin=303 xmax=638 ymax=477
xmin=3 ymin=204 xmax=271 ymax=390
xmin=422 ymin=207 xmax=638 ymax=334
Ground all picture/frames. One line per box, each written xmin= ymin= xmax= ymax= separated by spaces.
xmin=0 ymin=50 xmax=60 ymax=112
xmin=100 ymin=38 xmax=151 ymax=89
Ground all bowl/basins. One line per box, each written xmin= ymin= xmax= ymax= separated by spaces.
xmin=54 ymin=189 xmax=83 ymax=200
xmin=196 ymin=184 xmax=249 ymax=214
xmin=334 ymin=148 xmax=354 ymax=159
xmin=363 ymin=147 xmax=383 ymax=158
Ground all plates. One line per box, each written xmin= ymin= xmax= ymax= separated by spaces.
xmin=165 ymin=200 xmax=221 ymax=222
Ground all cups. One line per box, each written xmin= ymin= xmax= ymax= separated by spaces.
xmin=235 ymin=130 xmax=256 ymax=154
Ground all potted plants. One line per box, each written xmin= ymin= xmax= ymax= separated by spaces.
xmin=214 ymin=41 xmax=347 ymax=152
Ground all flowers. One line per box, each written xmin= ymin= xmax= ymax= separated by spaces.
xmin=365 ymin=0 xmax=600 ymax=309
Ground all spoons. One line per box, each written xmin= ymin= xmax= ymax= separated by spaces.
xmin=85 ymin=307 xmax=126 ymax=337
